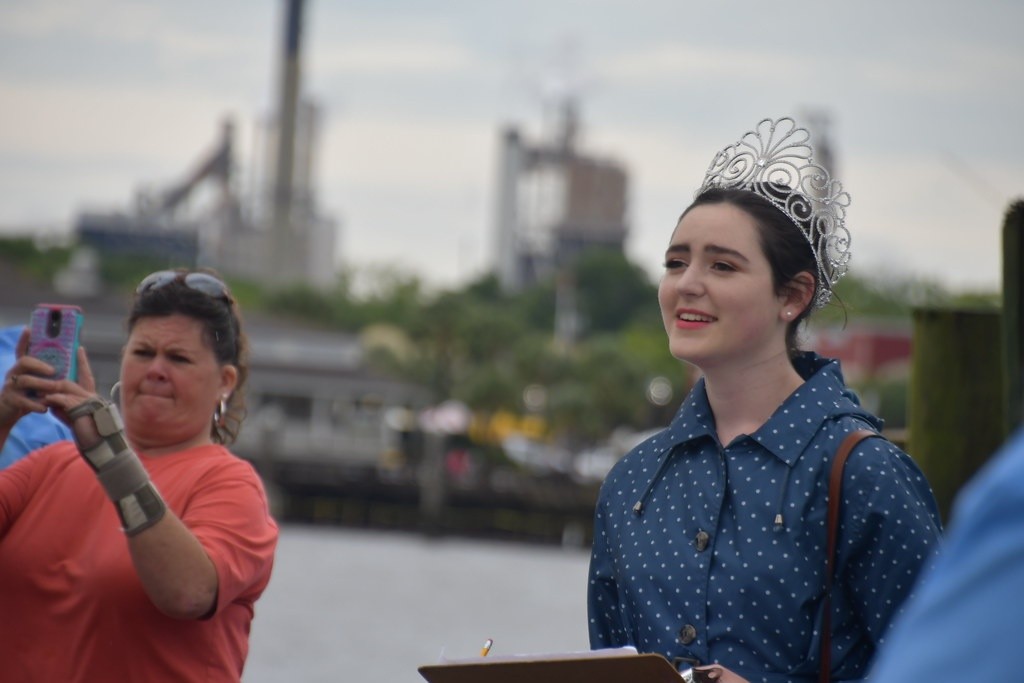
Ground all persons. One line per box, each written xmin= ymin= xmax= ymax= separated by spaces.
xmin=866 ymin=425 xmax=1024 ymax=682
xmin=587 ymin=117 xmax=943 ymax=683
xmin=1 ymin=269 xmax=280 ymax=683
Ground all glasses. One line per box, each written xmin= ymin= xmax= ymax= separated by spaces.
xmin=134 ymin=268 xmax=239 ymax=358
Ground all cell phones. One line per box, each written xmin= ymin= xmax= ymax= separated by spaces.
xmin=28 ymin=304 xmax=84 ymax=401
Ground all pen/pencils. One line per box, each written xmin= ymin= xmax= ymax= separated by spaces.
xmin=480 ymin=639 xmax=494 ymax=657
